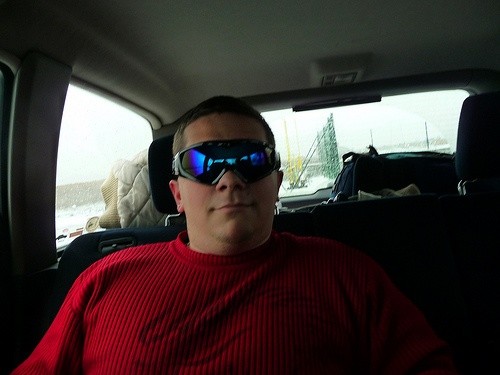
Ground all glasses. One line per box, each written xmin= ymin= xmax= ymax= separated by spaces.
xmin=169 ymin=138 xmax=281 ymax=185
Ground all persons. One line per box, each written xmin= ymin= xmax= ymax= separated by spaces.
xmin=10 ymin=95 xmax=457 ymax=374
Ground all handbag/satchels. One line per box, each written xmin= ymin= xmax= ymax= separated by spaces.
xmin=329 ymin=145 xmax=457 ymax=200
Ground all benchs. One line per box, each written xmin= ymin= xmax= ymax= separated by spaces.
xmin=34 ymin=89 xmax=500 ymax=375
